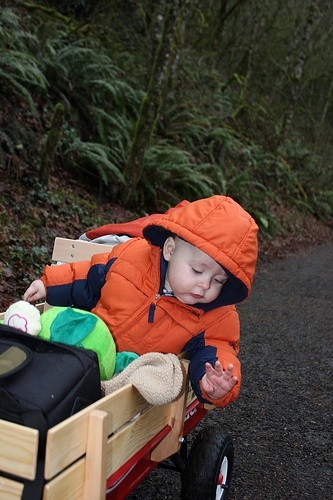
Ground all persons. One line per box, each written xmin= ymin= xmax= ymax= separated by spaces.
xmin=25 ymin=194 xmax=260 ymax=409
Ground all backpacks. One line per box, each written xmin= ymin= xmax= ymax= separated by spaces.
xmin=0 ymin=323 xmax=101 ymax=433
